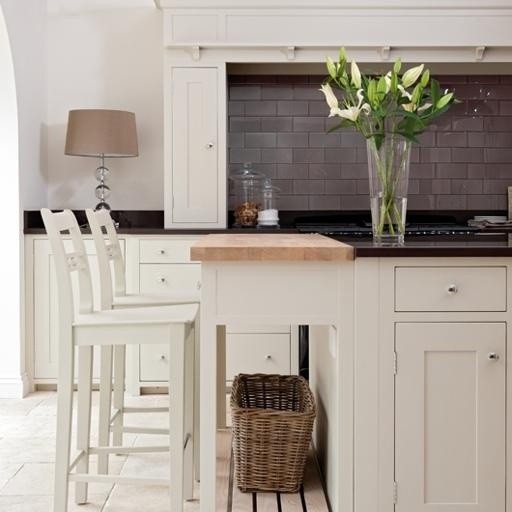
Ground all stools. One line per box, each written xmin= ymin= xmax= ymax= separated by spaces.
xmin=40 ymin=209 xmax=198 ymax=512
xmin=83 ymin=210 xmax=200 ymax=482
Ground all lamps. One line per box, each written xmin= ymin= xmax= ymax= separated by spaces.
xmin=64 ymin=108 xmax=141 ymax=231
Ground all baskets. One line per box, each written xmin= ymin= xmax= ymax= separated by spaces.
xmin=230 ymin=373 xmax=316 ymax=493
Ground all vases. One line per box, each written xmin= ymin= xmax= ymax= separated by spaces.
xmin=365 ymin=134 xmax=413 ymax=247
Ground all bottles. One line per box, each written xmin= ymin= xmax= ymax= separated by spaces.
xmin=228 ymin=162 xmax=281 ymax=230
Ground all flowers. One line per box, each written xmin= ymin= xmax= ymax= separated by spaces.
xmin=318 ymin=48 xmax=459 ymax=234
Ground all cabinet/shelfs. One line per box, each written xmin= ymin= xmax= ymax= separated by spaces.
xmin=394 ymin=265 xmax=508 ymax=512
xmin=170 ymin=66 xmax=219 ymax=224
xmin=32 ymin=238 xmax=126 ymax=380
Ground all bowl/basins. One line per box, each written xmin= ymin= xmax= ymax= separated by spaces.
xmin=474 ymin=214 xmax=507 ymax=223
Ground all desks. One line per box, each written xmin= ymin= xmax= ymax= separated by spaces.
xmin=189 ymin=232 xmax=356 ymax=512
